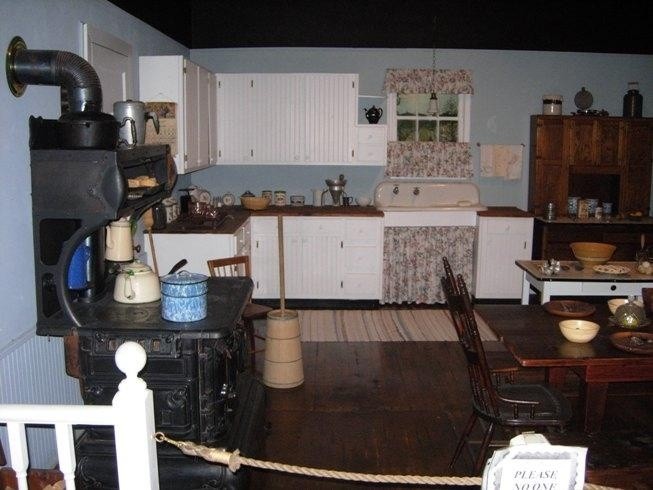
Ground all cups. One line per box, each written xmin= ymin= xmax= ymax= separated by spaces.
xmin=342 ymin=196 xmax=352 ymax=207
xmin=324 ymin=178 xmax=346 ymax=206
xmin=312 ymin=192 xmax=325 ymax=207
xmin=569 ymin=197 xmax=613 ymax=219
xmin=273 ymin=191 xmax=286 ymax=208
xmin=261 ymin=191 xmax=272 ymax=207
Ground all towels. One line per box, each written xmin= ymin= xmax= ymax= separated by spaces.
xmin=480 ymin=145 xmax=522 ymax=182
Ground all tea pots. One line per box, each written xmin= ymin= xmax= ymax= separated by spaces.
xmin=105 ymin=217 xmax=135 ymax=263
xmin=110 ymin=260 xmax=160 ymax=305
xmin=543 ymin=200 xmax=558 ymax=220
xmin=362 ymin=104 xmax=383 ymax=124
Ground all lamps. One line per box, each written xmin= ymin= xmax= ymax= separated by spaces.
xmin=426 ymin=12 xmax=441 ymax=116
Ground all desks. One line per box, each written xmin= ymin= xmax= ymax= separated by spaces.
xmin=472 ymin=299 xmax=652 ymax=457
xmin=514 ymin=258 xmax=652 ymax=304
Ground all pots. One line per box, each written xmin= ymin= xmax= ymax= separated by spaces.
xmin=57 ymin=112 xmax=139 ymax=150
xmin=111 ymin=98 xmax=160 ymax=150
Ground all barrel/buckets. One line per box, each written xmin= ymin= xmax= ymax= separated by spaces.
xmin=622 ymin=80 xmax=644 ymax=118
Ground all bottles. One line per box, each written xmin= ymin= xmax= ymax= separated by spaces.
xmin=59 ymin=240 xmax=90 ymax=290
xmin=150 ymin=197 xmax=178 ymax=230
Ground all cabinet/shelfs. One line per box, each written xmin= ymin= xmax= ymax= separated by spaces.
xmin=209 ymin=72 xmax=217 ymax=168
xmin=281 ymin=215 xmax=382 ymax=308
xmin=471 ymin=204 xmax=538 ymax=305
xmin=528 ymin=116 xmax=652 ymax=261
xmin=357 ymin=93 xmax=388 ymax=166
xmin=249 ymin=73 xmax=356 ymax=164
xmin=218 ymin=72 xmax=247 ymax=163
xmin=140 ymin=54 xmax=208 ymax=173
xmin=143 ymin=216 xmax=252 ymax=281
xmin=251 ymin=215 xmax=279 ymax=300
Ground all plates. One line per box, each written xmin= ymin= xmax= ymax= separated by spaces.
xmin=542 ymin=300 xmax=594 ymax=317
xmin=573 ymin=90 xmax=593 ymax=109
xmin=609 ymin=331 xmax=652 ymax=356
xmin=593 ymin=265 xmax=631 ymax=275
xmin=607 ymin=316 xmax=651 ymax=330
xmin=186 ymin=184 xmax=234 ymax=207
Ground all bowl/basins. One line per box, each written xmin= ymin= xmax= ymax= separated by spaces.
xmin=559 ymin=320 xmax=600 ymax=343
xmin=354 ymin=197 xmax=372 ymax=208
xmin=606 ymin=298 xmax=644 ymax=316
xmin=542 ymin=94 xmax=563 ymax=115
xmin=239 ymin=196 xmax=270 ymax=211
xmin=568 ymin=242 xmax=615 ymax=266
xmin=557 ymin=342 xmax=597 ymax=358
xmin=290 ymin=196 xmax=305 ymax=206
xmin=629 ymin=211 xmax=644 ymax=220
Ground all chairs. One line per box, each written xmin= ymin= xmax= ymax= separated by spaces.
xmin=205 ymin=255 xmax=271 ymax=370
xmin=431 ymin=257 xmax=574 ymax=478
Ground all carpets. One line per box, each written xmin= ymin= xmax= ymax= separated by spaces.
xmin=297 ymin=309 xmax=499 ymax=342
